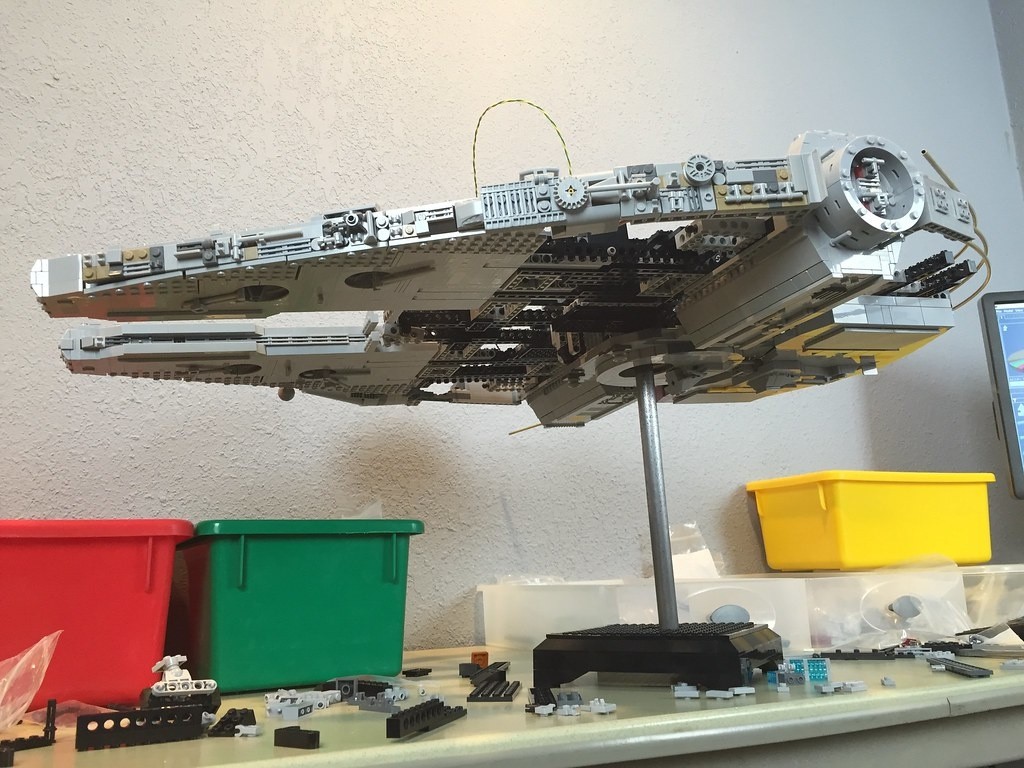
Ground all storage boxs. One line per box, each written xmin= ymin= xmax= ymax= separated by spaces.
xmin=0 ymin=518 xmax=194 ymax=712
xmin=165 ymin=519 xmax=425 ymax=693
xmin=746 ymin=470 xmax=998 ymax=573
xmin=476 ymin=564 xmax=1024 ymax=652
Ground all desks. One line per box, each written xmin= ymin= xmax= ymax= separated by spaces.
xmin=0 ymin=642 xmax=1024 ymax=768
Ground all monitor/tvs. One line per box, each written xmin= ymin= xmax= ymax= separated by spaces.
xmin=977 ymin=291 xmax=1024 ymax=500
